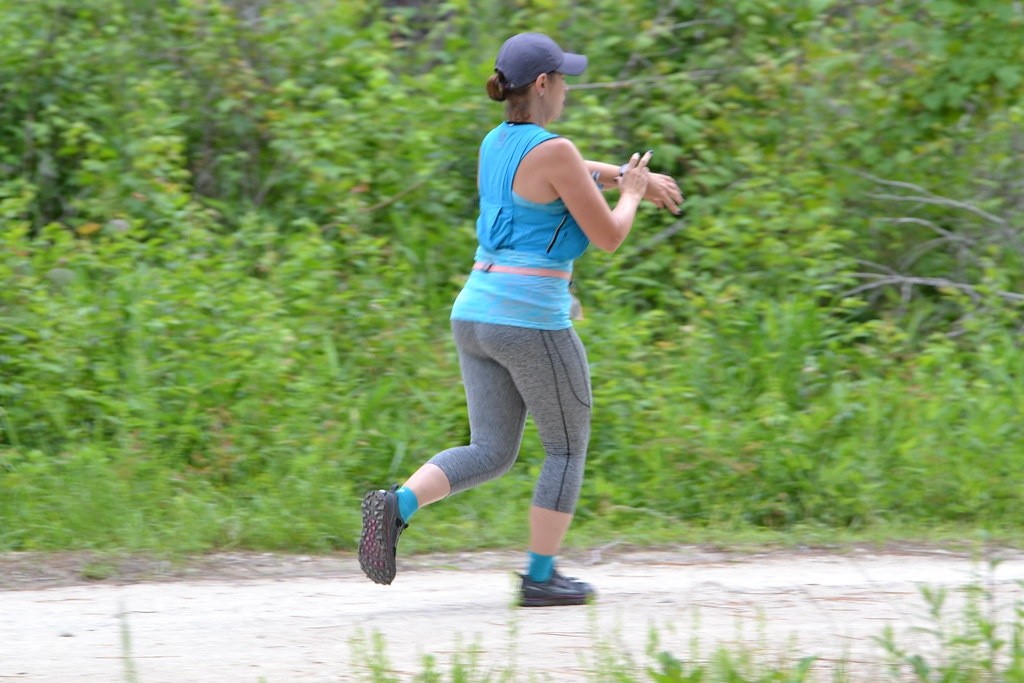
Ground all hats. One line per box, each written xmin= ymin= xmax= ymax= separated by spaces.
xmin=494 ymin=32 xmax=588 ymax=89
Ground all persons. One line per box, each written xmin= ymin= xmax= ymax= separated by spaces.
xmin=359 ymin=32 xmax=682 ymax=607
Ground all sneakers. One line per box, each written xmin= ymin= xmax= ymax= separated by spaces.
xmin=357 ymin=485 xmax=409 ymax=584
xmin=521 ymin=570 xmax=595 ymax=606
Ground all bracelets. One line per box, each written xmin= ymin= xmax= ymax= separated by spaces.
xmin=620 ymin=164 xmax=628 ymax=176
